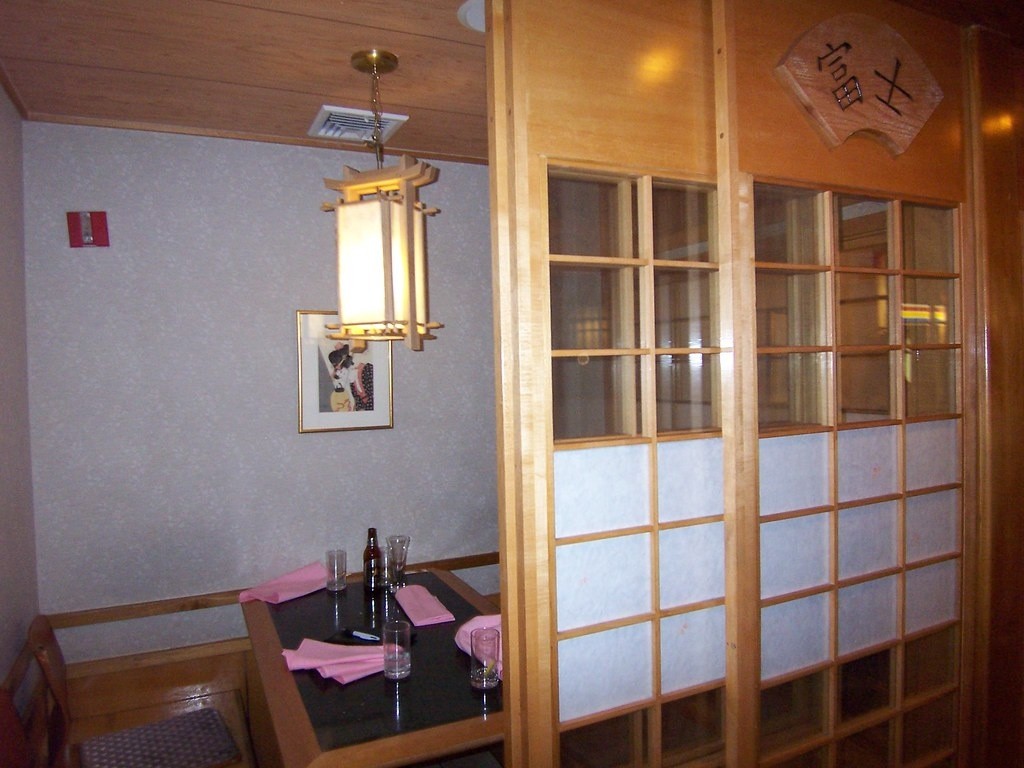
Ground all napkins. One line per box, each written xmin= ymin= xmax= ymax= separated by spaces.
xmin=454 ymin=614 xmax=503 ymax=681
xmin=281 ymin=637 xmax=405 ymax=685
xmin=238 ymin=561 xmax=334 ymax=604
xmin=394 ymin=585 xmax=455 ymax=626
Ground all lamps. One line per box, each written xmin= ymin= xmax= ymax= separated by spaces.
xmin=321 ymin=50 xmax=440 ymax=354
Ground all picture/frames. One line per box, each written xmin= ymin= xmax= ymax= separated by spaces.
xmin=296 ymin=310 xmax=393 ymax=433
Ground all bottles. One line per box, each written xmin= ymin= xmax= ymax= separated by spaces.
xmin=363 ymin=528 xmax=383 ymax=600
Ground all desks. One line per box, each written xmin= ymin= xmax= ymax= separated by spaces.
xmin=241 ymin=567 xmax=503 ymax=768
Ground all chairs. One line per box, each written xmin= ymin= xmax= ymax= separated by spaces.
xmin=0 ymin=615 xmax=256 ymax=768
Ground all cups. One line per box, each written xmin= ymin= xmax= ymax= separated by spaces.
xmin=471 ymin=628 xmax=500 ymax=689
xmin=384 ymin=621 xmax=411 ymax=679
xmin=326 ymin=549 xmax=347 ymax=591
xmin=381 ymin=535 xmax=411 ymax=593
